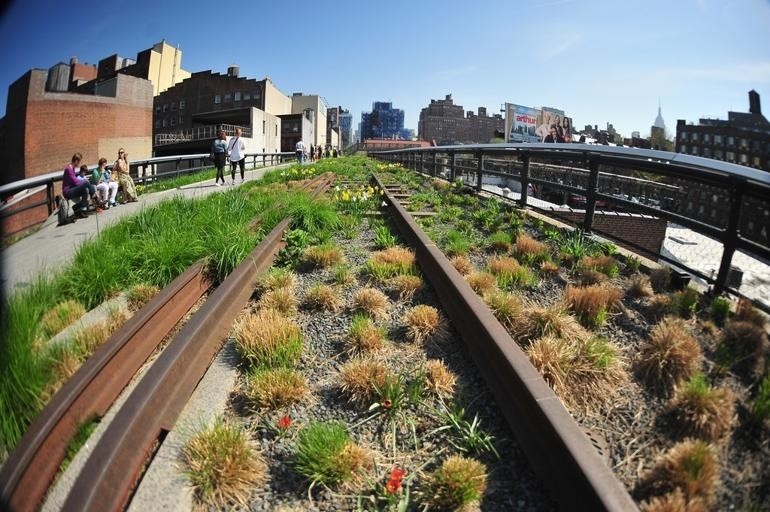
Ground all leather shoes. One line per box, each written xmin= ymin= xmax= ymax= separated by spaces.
xmin=78 ymin=213 xmax=88 ymax=218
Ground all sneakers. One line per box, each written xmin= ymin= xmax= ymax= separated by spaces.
xmin=96 ymin=198 xmax=138 ymax=212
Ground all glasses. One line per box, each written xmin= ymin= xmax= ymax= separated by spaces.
xmin=119 ymin=152 xmax=124 ymax=154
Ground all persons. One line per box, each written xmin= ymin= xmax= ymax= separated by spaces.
xmin=210 ymin=131 xmax=230 ymax=186
xmin=295 ymin=138 xmax=304 ymax=163
xmin=534 ymin=112 xmax=571 ymax=143
xmin=76 ymin=165 xmax=97 ymax=201
xmin=62 ymin=153 xmax=97 ymax=219
xmin=92 ymin=158 xmax=118 ymax=210
xmin=116 ymin=148 xmax=139 ymax=203
xmin=228 ymin=128 xmax=247 ymax=185
xmin=303 ymin=142 xmax=342 ymax=163
